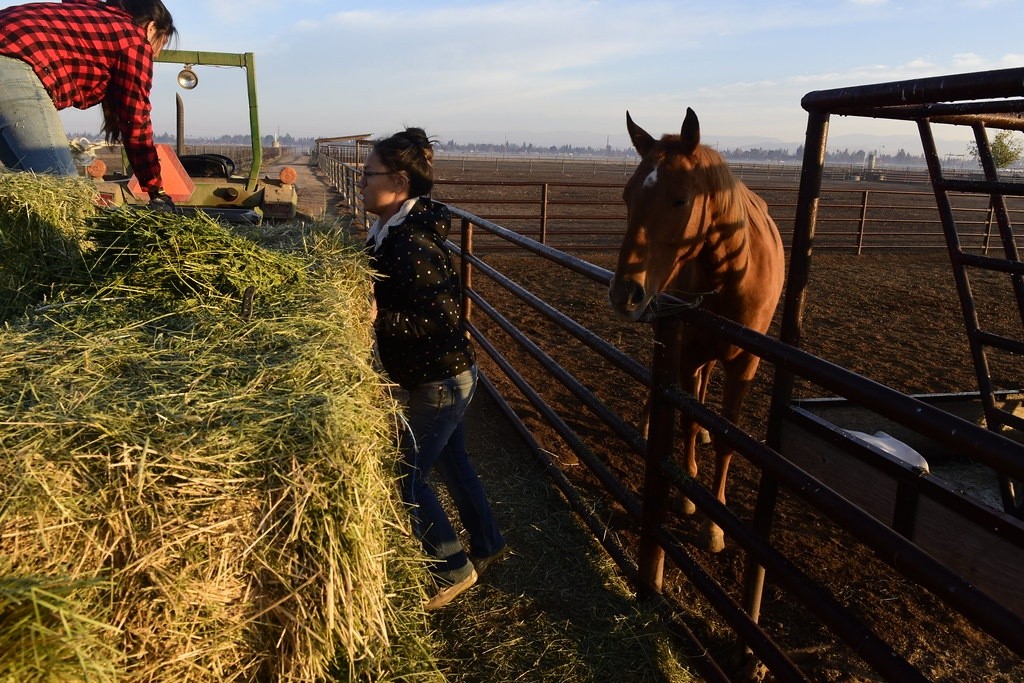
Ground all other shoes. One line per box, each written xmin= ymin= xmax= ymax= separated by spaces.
xmin=421 ymin=559 xmax=479 ymax=611
xmin=468 ymin=544 xmax=508 ymax=577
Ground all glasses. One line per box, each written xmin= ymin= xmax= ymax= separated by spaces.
xmin=363 ymin=170 xmax=410 ymax=187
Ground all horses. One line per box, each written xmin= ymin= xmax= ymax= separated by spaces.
xmin=607 ymin=105 xmax=786 ymax=554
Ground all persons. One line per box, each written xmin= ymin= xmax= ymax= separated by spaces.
xmin=357 ymin=127 xmax=507 ymax=614
xmin=1 ymin=0 xmax=177 ymax=215
